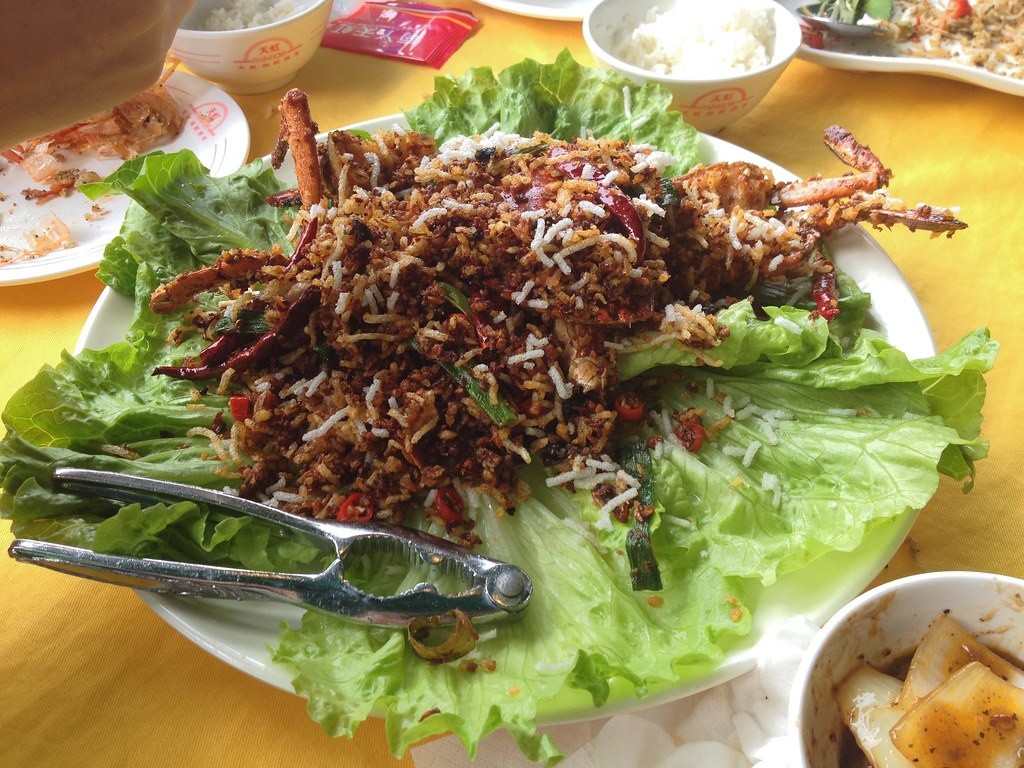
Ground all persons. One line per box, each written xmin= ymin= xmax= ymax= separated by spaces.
xmin=1 ymin=0 xmax=196 ymax=152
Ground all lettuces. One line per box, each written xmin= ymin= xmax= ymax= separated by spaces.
xmin=0 ymin=48 xmax=999 ymax=767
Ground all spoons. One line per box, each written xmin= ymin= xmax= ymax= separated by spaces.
xmin=801 ymin=8 xmax=884 ymax=39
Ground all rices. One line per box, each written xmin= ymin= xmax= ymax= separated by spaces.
xmin=605 ymin=1 xmax=779 ymax=82
xmin=190 ymin=1 xmax=314 ymax=31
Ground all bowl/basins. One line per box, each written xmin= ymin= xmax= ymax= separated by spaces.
xmin=794 ymin=570 xmax=1024 ymax=767
xmin=584 ymin=1 xmax=804 ymax=140
xmin=168 ymin=3 xmax=332 ymax=94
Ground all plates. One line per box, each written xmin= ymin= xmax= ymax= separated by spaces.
xmin=1 ymin=69 xmax=250 ymax=291
xmin=784 ymin=2 xmax=1024 ymax=102
xmin=68 ymin=111 xmax=942 ymax=725
